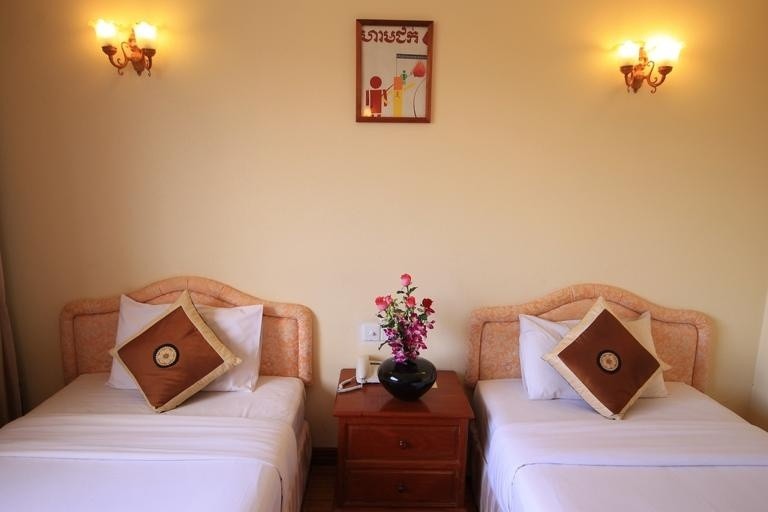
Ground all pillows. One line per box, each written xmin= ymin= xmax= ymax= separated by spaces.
xmin=519 ymin=312 xmax=672 ymax=397
xmin=110 ymin=290 xmax=242 ymax=412
xmin=107 ymin=292 xmax=264 ymax=395
xmin=544 ymin=296 xmax=656 ymax=420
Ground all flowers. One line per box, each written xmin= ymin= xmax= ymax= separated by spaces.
xmin=376 ymin=274 xmax=437 ymax=363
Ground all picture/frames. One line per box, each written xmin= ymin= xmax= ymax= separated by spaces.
xmin=356 ymin=19 xmax=433 ymax=124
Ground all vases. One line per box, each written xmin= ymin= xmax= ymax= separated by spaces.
xmin=376 ymin=356 xmax=436 ymax=402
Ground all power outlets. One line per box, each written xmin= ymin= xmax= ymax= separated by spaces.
xmin=360 ymin=322 xmax=381 ymax=344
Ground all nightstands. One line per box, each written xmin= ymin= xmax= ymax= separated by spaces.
xmin=331 ymin=369 xmax=474 ymax=512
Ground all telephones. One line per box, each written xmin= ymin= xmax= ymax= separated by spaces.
xmin=337 ymin=354 xmax=382 ymax=393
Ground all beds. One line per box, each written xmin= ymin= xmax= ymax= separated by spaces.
xmin=467 ymin=283 xmax=768 ymax=512
xmin=0 ymin=276 xmax=312 ymax=511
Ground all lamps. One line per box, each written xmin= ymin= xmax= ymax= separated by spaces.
xmin=94 ymin=18 xmax=162 ymax=76
xmin=617 ymin=33 xmax=684 ymax=94
